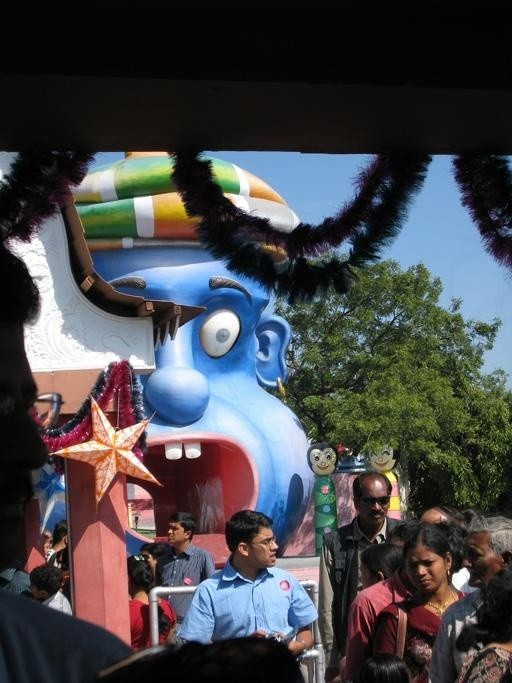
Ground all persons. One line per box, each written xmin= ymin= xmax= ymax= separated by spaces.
xmin=175 ymin=507 xmax=319 ymax=661
xmin=1 ymin=242 xmax=137 ymax=679
xmin=330 ymin=501 xmax=512 ymax=683
xmin=13 ymin=508 xmax=218 ymax=651
xmin=315 ymin=469 xmax=408 ymax=683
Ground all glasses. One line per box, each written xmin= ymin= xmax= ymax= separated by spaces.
xmin=361 ymin=496 xmax=389 ymax=504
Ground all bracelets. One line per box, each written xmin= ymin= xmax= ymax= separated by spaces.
xmin=297 ymin=639 xmax=307 ymax=654
